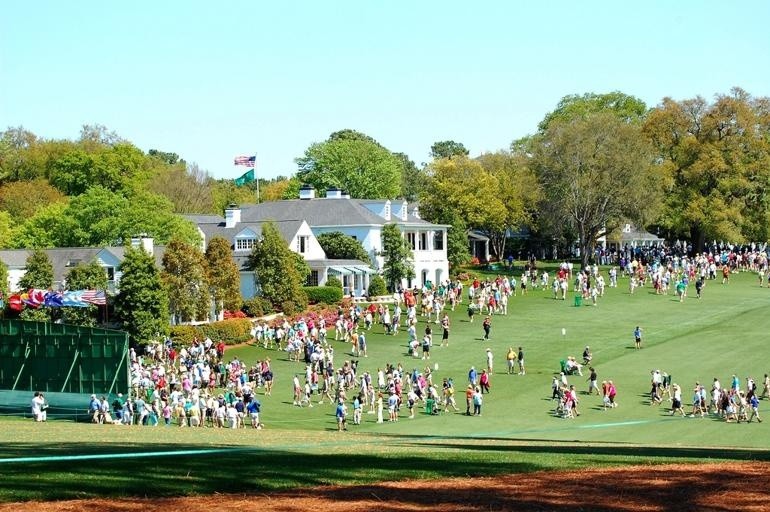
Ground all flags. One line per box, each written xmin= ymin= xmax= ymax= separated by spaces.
xmin=234 ymin=169 xmax=254 ymax=187
xmin=234 ymin=156 xmax=256 ymax=167
xmin=0 ymin=289 xmax=107 ymax=311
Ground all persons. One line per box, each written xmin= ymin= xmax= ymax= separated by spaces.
xmin=88 ymin=241 xmax=770 ymax=434
xmin=32 ymin=392 xmax=47 ymax=423
